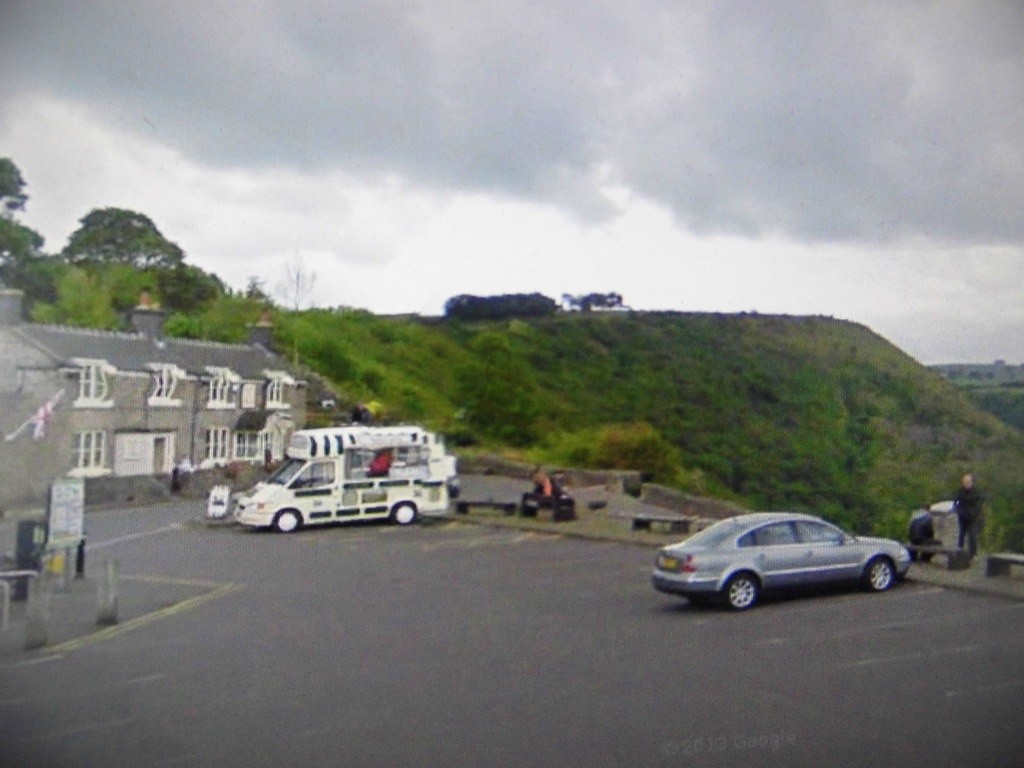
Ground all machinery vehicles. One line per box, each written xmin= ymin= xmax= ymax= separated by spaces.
xmin=234 ymin=428 xmax=457 ymax=534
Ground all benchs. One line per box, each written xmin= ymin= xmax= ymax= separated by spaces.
xmin=906 ymin=544 xmax=970 ymax=572
xmin=986 ymin=552 xmax=1024 ymax=577
xmin=449 ymin=500 xmax=517 ymax=517
xmin=619 ymin=513 xmax=700 ymax=534
xmin=521 ymin=491 xmax=576 ymax=521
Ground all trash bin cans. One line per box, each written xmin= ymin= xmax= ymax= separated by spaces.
xmin=15 ymin=511 xmax=48 ymax=567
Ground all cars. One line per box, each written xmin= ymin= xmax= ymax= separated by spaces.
xmin=651 ymin=513 xmax=910 ymax=611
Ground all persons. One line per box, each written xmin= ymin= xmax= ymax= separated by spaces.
xmin=952 ymin=473 xmax=987 ymax=555
xmin=908 ymin=503 xmax=942 ymax=561
xmin=529 ymin=467 xmax=578 ymax=519
